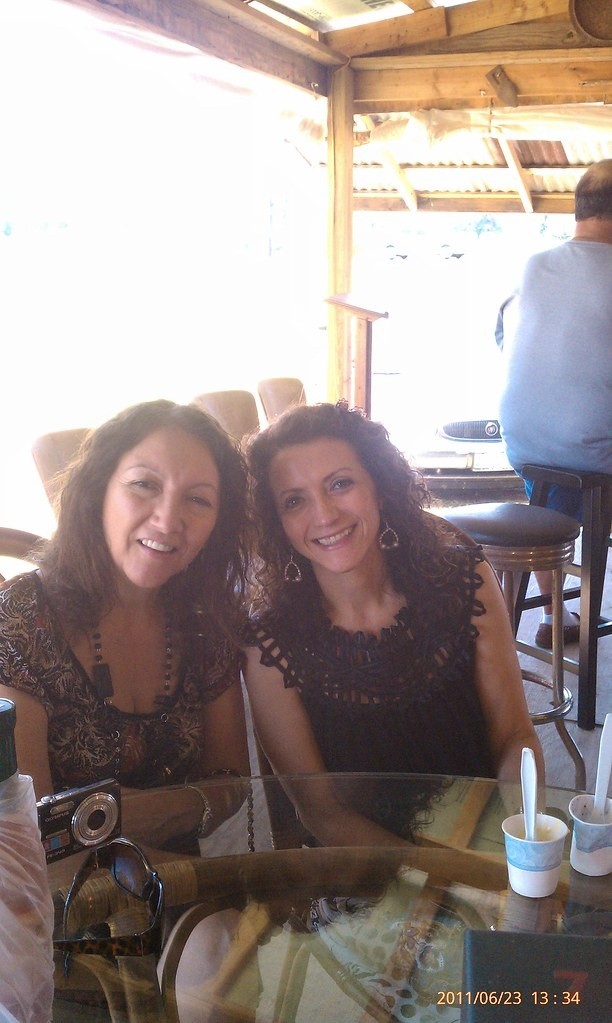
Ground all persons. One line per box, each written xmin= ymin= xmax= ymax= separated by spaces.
xmin=0 ymin=400 xmax=264 ymax=1022
xmin=499 ymin=159 xmax=612 ymax=647
xmin=243 ymin=399 xmax=571 ymax=1009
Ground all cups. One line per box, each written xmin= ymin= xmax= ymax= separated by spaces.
xmin=569 ymin=795 xmax=612 ymax=876
xmin=501 ymin=814 xmax=569 ymax=898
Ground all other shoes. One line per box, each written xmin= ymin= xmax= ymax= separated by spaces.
xmin=535 ymin=611 xmax=580 ymax=648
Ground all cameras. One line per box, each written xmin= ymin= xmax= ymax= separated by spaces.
xmin=35 ymin=778 xmax=123 ymax=865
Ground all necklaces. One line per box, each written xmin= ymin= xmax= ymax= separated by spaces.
xmin=91 ymin=611 xmax=176 ymax=788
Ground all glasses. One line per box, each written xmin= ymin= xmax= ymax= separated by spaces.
xmin=52 ymin=837 xmax=164 ymax=979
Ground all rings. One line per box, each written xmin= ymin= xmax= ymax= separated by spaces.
xmin=489 ymin=924 xmax=497 ymax=931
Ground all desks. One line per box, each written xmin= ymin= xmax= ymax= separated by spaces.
xmin=47 ymin=770 xmax=611 ymax=1023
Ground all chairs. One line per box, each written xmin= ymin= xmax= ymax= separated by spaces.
xmin=197 ymin=390 xmax=260 ymax=442
xmin=256 ymin=733 xmax=400 ymax=1023
xmin=258 ymin=377 xmax=306 ymax=424
xmin=33 ymin=427 xmax=92 ymax=513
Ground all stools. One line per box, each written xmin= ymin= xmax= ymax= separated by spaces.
xmin=512 ymin=464 xmax=612 ymax=730
xmin=426 ymin=502 xmax=586 ymax=794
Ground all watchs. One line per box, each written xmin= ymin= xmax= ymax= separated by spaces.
xmin=185 ymin=785 xmax=214 ymax=839
